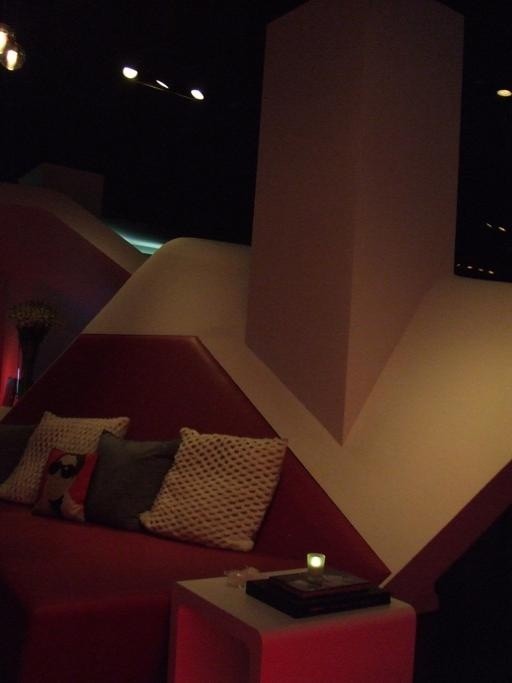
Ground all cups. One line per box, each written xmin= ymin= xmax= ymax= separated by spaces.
xmin=307 ymin=553 xmax=326 ymax=583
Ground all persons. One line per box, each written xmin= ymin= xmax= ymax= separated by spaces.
xmin=44 ymin=450 xmax=86 ymax=518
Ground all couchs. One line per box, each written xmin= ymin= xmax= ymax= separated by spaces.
xmin=1 ymin=334 xmax=390 ymax=682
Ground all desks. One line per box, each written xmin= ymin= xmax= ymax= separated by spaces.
xmin=171 ymin=568 xmax=417 ymax=683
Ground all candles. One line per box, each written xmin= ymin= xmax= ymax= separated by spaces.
xmin=306 ymin=552 xmax=326 ymax=578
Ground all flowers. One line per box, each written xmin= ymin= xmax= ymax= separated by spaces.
xmin=7 ymin=293 xmax=62 ymax=400
